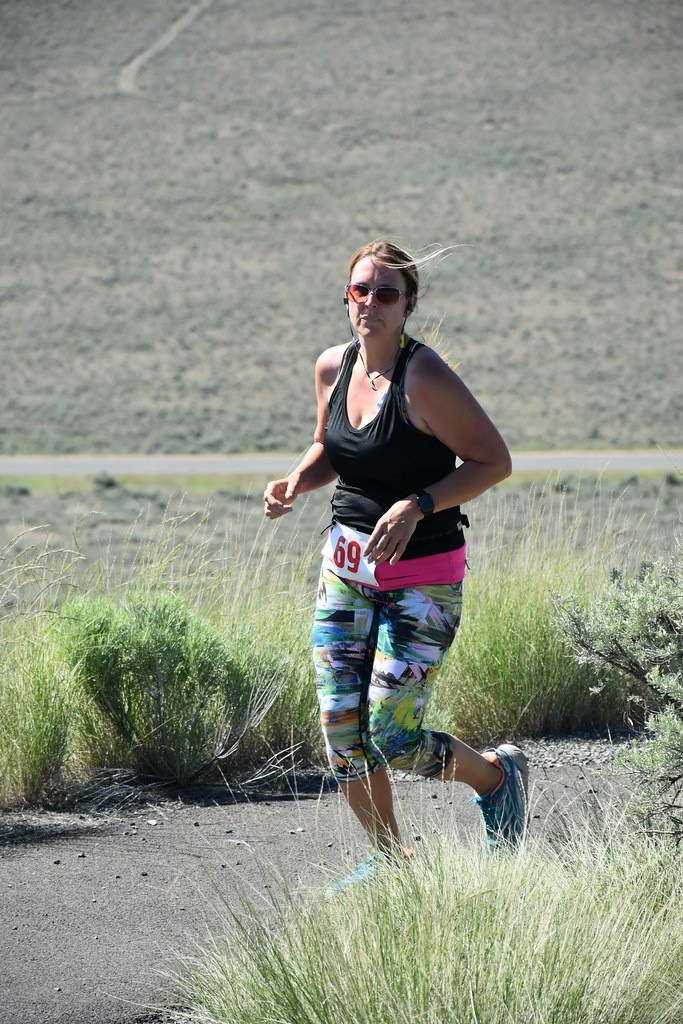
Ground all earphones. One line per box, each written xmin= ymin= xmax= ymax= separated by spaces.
xmin=343 ymin=297 xmax=348 ymax=304
xmin=406 ymin=301 xmax=415 ymax=312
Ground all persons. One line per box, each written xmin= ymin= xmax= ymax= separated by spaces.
xmin=262 ymin=240 xmax=531 ymax=896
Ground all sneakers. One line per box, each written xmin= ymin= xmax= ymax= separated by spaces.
xmin=324 ymin=847 xmax=413 ymax=903
xmin=470 ymin=744 xmax=531 ymax=855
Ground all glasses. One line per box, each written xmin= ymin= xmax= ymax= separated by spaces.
xmin=344 ymin=284 xmax=408 ymax=305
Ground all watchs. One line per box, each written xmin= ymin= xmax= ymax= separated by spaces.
xmin=413 ymin=488 xmax=436 ymax=519
